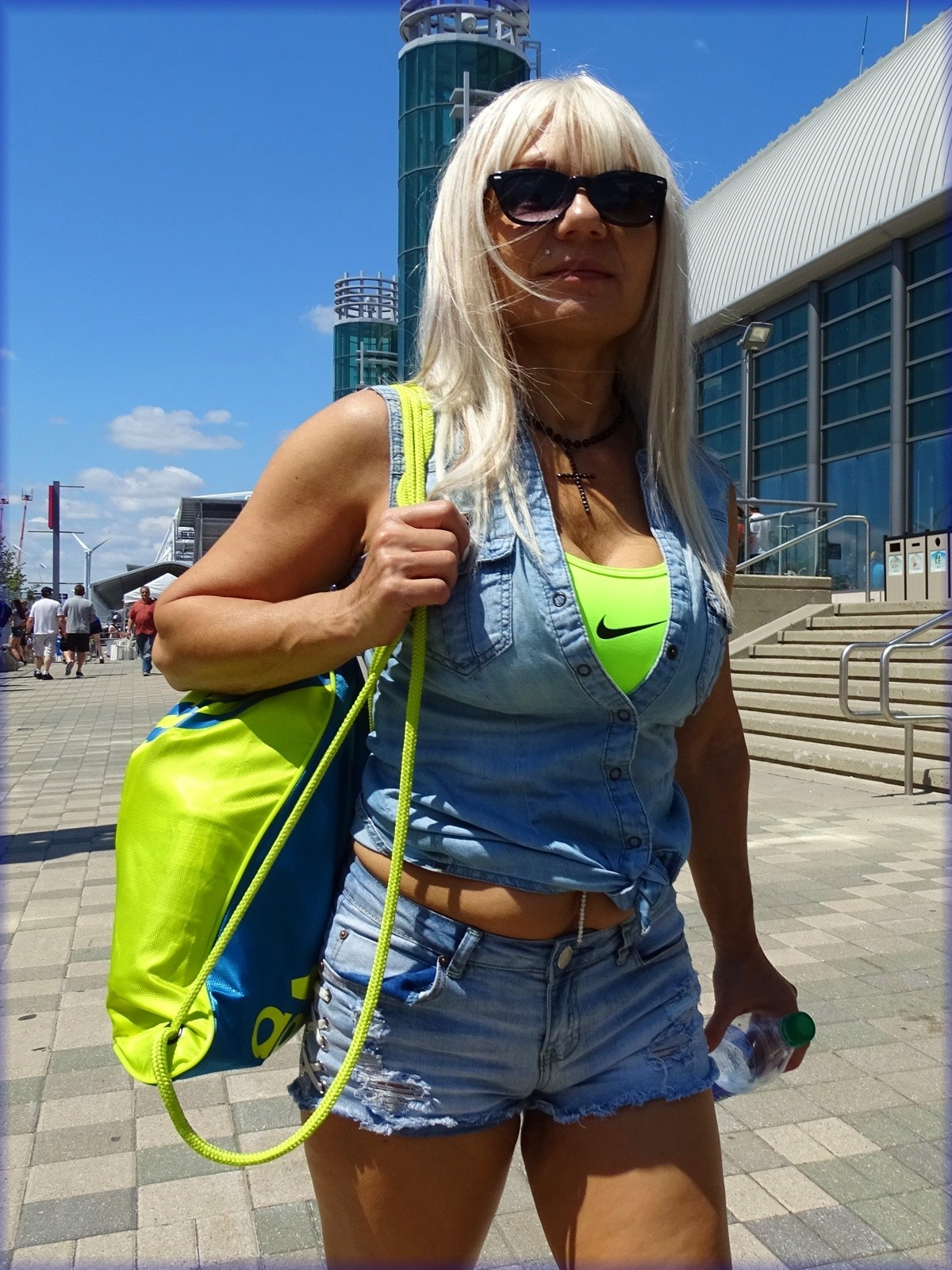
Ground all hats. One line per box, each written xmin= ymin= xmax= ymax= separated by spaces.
xmin=747 ymin=497 xmax=760 ymax=509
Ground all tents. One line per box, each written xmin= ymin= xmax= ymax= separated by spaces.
xmin=121 ymin=572 xmax=178 ymax=637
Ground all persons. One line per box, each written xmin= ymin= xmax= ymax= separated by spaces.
xmin=746 ymin=497 xmax=772 ymax=574
xmin=108 ymin=618 xmax=120 ymax=638
xmin=737 ymin=505 xmax=755 ymax=574
xmin=89 ymin=614 xmax=104 ymax=664
xmin=150 ymin=73 xmax=810 ymax=1270
xmin=25 ymin=586 xmax=66 ymax=681
xmin=8 ymin=598 xmax=28 ymax=668
xmin=123 ymin=586 xmax=157 ymax=676
xmin=59 ymin=585 xmax=96 ymax=677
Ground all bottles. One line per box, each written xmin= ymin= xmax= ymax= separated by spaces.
xmin=711 ymin=1012 xmax=816 ymax=1104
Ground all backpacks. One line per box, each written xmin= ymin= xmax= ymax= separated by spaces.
xmin=102 ymin=378 xmax=436 ymax=1169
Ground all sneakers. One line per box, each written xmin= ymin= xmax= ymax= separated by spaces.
xmin=143 ymin=672 xmax=151 ymax=676
xmin=76 ymin=660 xmax=86 ymax=664
xmin=99 ymin=656 xmax=104 ymax=664
xmin=65 ymin=660 xmax=74 ymax=676
xmin=34 ymin=670 xmax=42 ymax=680
xmin=76 ymin=671 xmax=84 ymax=676
xmin=42 ymin=673 xmax=54 ymax=681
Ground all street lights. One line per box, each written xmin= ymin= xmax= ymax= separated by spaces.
xmin=735 ymin=323 xmax=774 ymax=575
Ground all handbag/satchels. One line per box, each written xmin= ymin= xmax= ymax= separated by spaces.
xmin=10 ymin=626 xmax=22 ymax=634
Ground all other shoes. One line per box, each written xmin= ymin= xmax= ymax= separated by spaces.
xmin=22 ymin=661 xmax=27 ymax=666
xmin=18 ymin=662 xmax=24 ymax=667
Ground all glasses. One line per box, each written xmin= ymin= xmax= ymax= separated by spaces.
xmin=481 ymin=168 xmax=670 ymax=227
xmin=141 ymin=592 xmax=149 ymax=593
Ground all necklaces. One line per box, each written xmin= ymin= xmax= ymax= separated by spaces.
xmin=522 ymin=404 xmax=633 ymax=513
